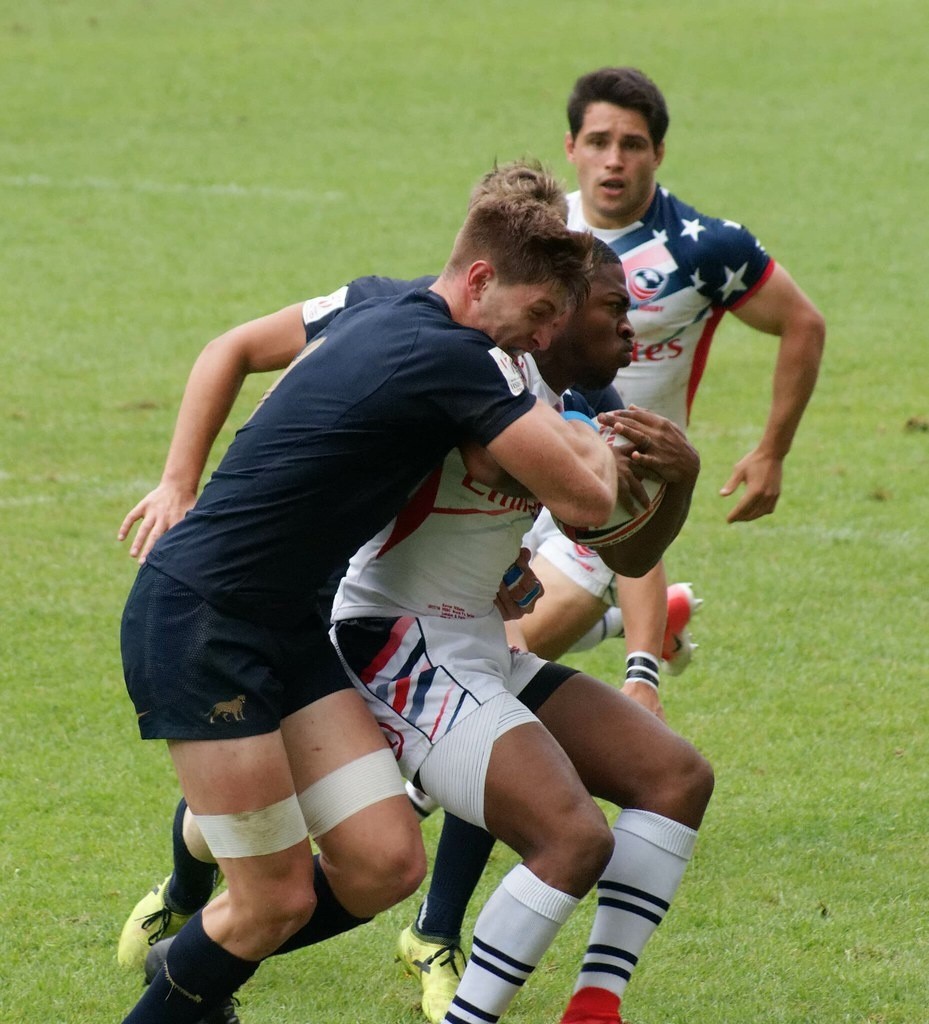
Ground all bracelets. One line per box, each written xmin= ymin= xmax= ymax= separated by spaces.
xmin=624 ymin=651 xmax=660 ymax=692
xmin=561 ymin=411 xmax=598 ymax=432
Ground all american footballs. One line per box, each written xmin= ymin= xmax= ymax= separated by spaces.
xmin=552 ymin=406 xmax=668 ymax=549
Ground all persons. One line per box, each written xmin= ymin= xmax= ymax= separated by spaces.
xmin=113 ymin=68 xmax=824 ymax=1024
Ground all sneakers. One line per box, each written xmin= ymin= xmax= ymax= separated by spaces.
xmin=393 ymin=922 xmax=467 ymax=1024
xmin=660 ymin=582 xmax=700 ymax=676
xmin=117 ymin=870 xmax=226 ymax=974
xmin=141 ymin=934 xmax=240 ymax=1024
